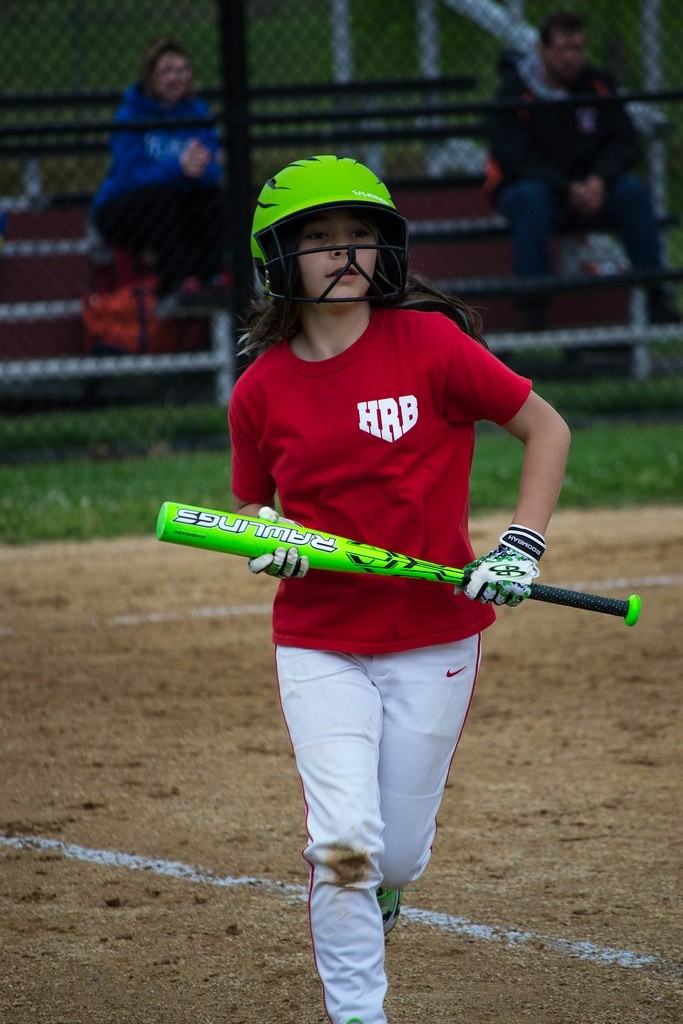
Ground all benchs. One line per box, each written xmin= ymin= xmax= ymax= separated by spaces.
xmin=0 ymin=79 xmax=666 ymax=405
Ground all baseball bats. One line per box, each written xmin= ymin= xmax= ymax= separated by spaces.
xmin=154 ymin=500 xmax=642 ymax=629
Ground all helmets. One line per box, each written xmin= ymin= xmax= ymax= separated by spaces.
xmin=249 ymin=155 xmax=413 ymax=305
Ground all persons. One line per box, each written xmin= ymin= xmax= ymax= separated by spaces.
xmin=229 ymin=153 xmax=571 ymax=1023
xmin=493 ymin=13 xmax=682 ymax=330
xmin=90 ymin=39 xmax=258 ymax=304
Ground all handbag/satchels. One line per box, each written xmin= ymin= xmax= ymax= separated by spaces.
xmin=84 ymin=265 xmax=172 ymax=358
xmin=560 ymin=198 xmax=632 ymax=289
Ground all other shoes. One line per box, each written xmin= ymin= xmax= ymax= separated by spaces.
xmin=375 ymin=886 xmax=401 ymax=935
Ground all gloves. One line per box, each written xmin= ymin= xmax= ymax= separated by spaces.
xmin=452 ymin=524 xmax=547 ymax=607
xmin=247 ymin=505 xmax=309 ymax=580
